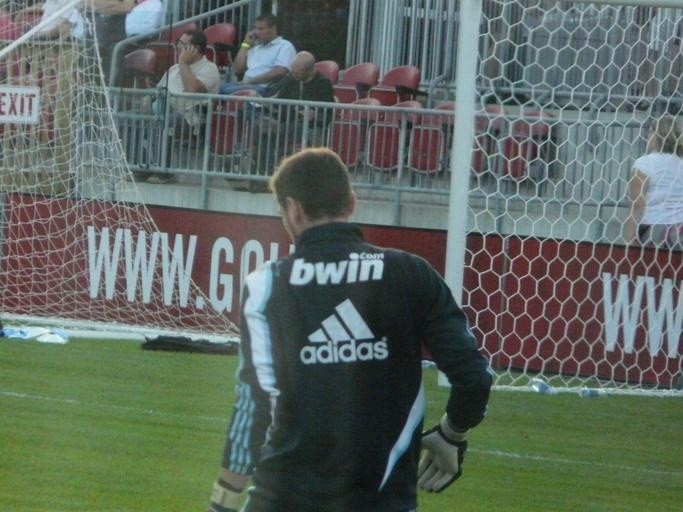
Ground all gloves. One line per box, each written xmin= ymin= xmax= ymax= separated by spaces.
xmin=416 ymin=413 xmax=470 ymax=494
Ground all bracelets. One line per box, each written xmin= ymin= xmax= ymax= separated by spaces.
xmin=239 ymin=42 xmax=250 ymax=51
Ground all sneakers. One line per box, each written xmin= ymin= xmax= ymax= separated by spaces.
xmin=125 ymin=169 xmax=176 ymax=184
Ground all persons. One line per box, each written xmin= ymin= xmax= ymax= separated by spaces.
xmin=216 ymin=13 xmax=297 ymax=111
xmin=244 ymin=52 xmax=335 ymax=192
xmin=208 ymin=146 xmax=492 ymax=511
xmin=18 ymin=0 xmax=169 ymax=86
xmin=125 ymin=29 xmax=219 ymax=184
xmin=621 ymin=114 xmax=683 ymax=250
